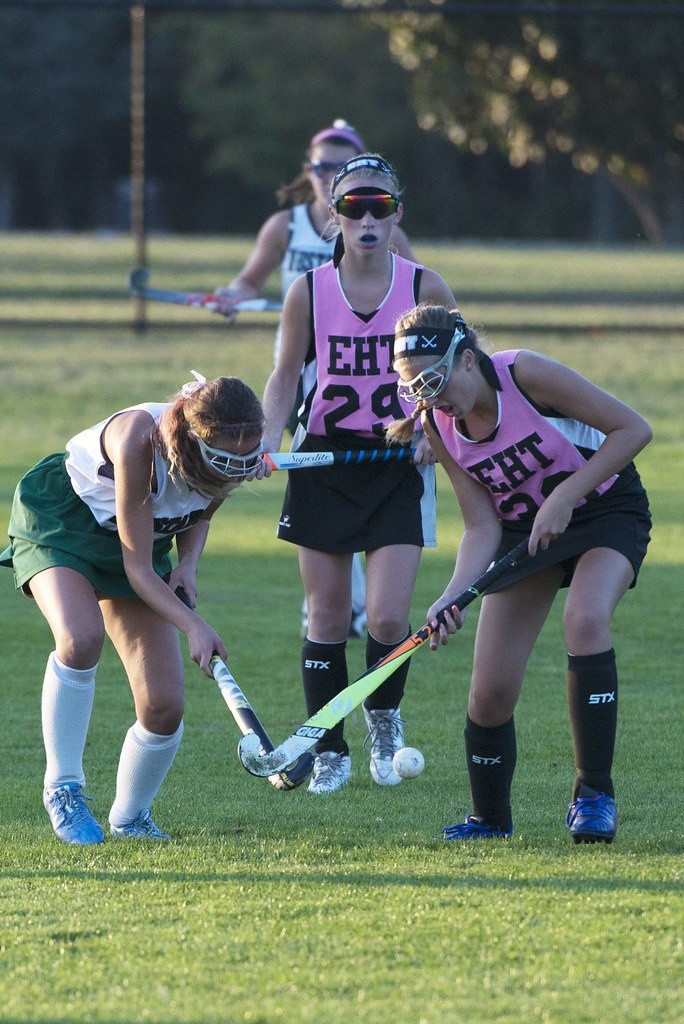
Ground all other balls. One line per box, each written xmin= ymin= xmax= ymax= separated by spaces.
xmin=391 ymin=747 xmax=427 ymax=779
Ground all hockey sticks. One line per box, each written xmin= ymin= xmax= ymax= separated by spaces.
xmin=263 ymin=450 xmax=419 ymax=470
xmin=128 ymin=267 xmax=285 ymax=317
xmin=168 ymin=571 xmax=316 ymax=792
xmin=236 ymin=534 xmax=528 ymax=780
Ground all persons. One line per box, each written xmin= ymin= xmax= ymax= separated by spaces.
xmin=383 ymin=305 xmax=654 ymax=845
xmin=245 ymin=152 xmax=464 ymax=796
xmin=0 ymin=372 xmax=268 ymax=846
xmin=208 ymin=115 xmax=440 ymax=641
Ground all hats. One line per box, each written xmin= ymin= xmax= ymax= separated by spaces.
xmin=332 ymin=154 xmax=399 ymax=200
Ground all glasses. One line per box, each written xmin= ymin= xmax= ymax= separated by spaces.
xmin=397 ymin=327 xmax=467 ymax=404
xmin=309 ymin=158 xmax=345 ymax=178
xmin=333 ymin=194 xmax=398 ymax=219
xmin=193 ymin=432 xmax=264 ymax=478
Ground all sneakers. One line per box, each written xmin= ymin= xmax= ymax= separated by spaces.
xmin=110 ymin=808 xmax=172 ymax=842
xmin=361 ymin=700 xmax=406 ymax=786
xmin=566 ymin=788 xmax=619 ymax=842
xmin=43 ymin=782 xmax=104 ymax=845
xmin=440 ymin=815 xmax=514 ymax=843
xmin=307 ymin=750 xmax=351 ymax=794
